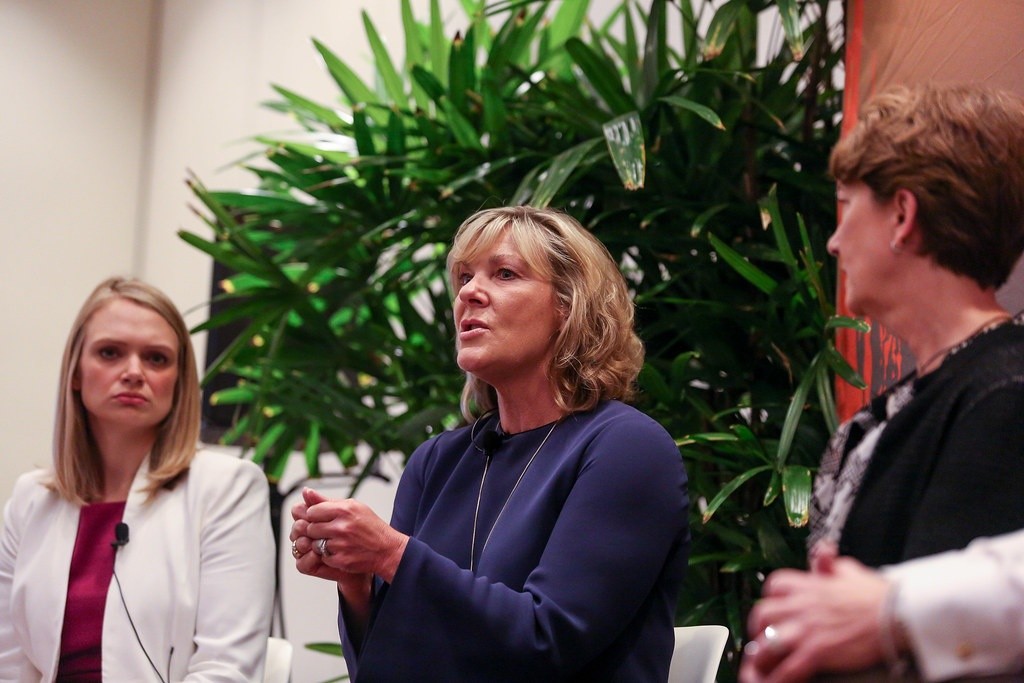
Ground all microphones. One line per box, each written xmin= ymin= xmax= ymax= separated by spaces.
xmin=110 ymin=522 xmax=130 ymax=547
xmin=483 ymin=431 xmax=501 ymax=457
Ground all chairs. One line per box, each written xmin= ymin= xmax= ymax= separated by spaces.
xmin=668 ymin=625 xmax=730 ymax=683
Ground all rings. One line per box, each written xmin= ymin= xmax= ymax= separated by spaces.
xmin=318 ymin=539 xmax=331 ymax=557
xmin=763 ymin=622 xmax=785 ymax=657
xmin=293 ymin=540 xmax=304 ymax=557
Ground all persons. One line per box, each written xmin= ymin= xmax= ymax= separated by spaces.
xmin=747 ymin=521 xmax=1024 ymax=683
xmin=291 ymin=207 xmax=691 ymax=682
xmin=1 ymin=276 xmax=275 ymax=683
xmin=814 ymin=79 xmax=1024 ymax=683
